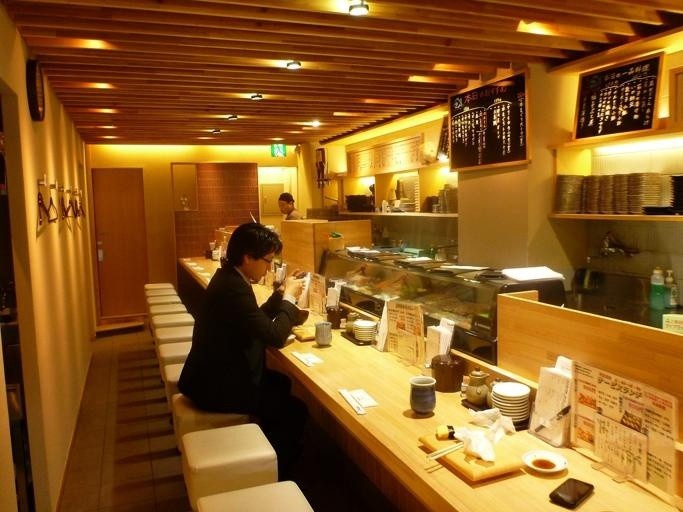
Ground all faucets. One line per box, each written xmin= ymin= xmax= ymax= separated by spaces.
xmin=587 ymin=233 xmax=619 ymax=262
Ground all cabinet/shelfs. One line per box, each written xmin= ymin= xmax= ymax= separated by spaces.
xmin=319 ymin=245 xmax=569 ymax=366
xmin=546 ymin=126 xmax=683 ymax=222
xmin=335 ymin=160 xmax=458 ymax=218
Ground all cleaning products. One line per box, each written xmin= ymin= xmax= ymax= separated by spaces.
xmin=649 ymin=266 xmax=665 ymax=309
xmin=666 ymin=269 xmax=679 ymax=308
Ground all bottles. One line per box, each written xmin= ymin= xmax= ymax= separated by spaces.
xmin=649 ymin=266 xmax=679 ymax=310
xmin=465 ymin=368 xmax=489 ymax=407
xmin=345 ymin=313 xmax=360 ymax=334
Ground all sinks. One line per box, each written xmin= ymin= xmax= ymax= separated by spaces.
xmin=565 ymin=292 xmax=633 ymax=317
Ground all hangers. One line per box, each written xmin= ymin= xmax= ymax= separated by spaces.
xmin=37 ymin=180 xmax=86 ymax=226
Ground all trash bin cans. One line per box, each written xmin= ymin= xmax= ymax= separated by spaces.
xmin=355 ymin=301 xmax=374 ymax=311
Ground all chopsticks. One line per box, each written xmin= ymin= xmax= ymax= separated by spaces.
xmin=425 ymin=441 xmax=465 ymax=462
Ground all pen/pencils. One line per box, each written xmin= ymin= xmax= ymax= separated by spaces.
xmin=532 ymin=406 xmax=569 ymax=433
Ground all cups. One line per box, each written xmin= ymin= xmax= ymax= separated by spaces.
xmin=409 ymin=376 xmax=436 ymax=415
xmin=314 ymin=321 xmax=333 ymax=346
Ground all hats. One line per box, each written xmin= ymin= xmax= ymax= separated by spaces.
xmin=279 ymin=193 xmax=294 ymax=202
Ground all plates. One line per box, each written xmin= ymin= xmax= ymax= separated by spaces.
xmin=556 ymin=171 xmax=683 ymax=215
xmin=414 ymin=182 xmax=420 ymax=212
xmin=354 ymin=320 xmax=377 ymax=341
xmin=348 ymin=245 xmax=447 ymax=272
xmin=212 ymin=250 xmax=219 ymax=261
xmin=399 ymin=197 xmax=413 ymax=211
xmin=440 ymin=264 xmax=489 ymax=274
xmin=523 ymin=450 xmax=568 ymax=474
xmin=429 ymin=184 xmax=458 ymax=214
xmin=491 ymin=382 xmax=531 ymax=424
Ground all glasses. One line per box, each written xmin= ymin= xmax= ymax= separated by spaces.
xmin=260 ymin=256 xmax=273 ymax=264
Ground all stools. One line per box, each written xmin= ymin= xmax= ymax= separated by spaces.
xmin=145 ymin=283 xmax=313 ymax=512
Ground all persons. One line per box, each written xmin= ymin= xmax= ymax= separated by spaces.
xmin=279 ymin=193 xmax=304 ymax=220
xmin=176 ymin=221 xmax=308 ymax=413
